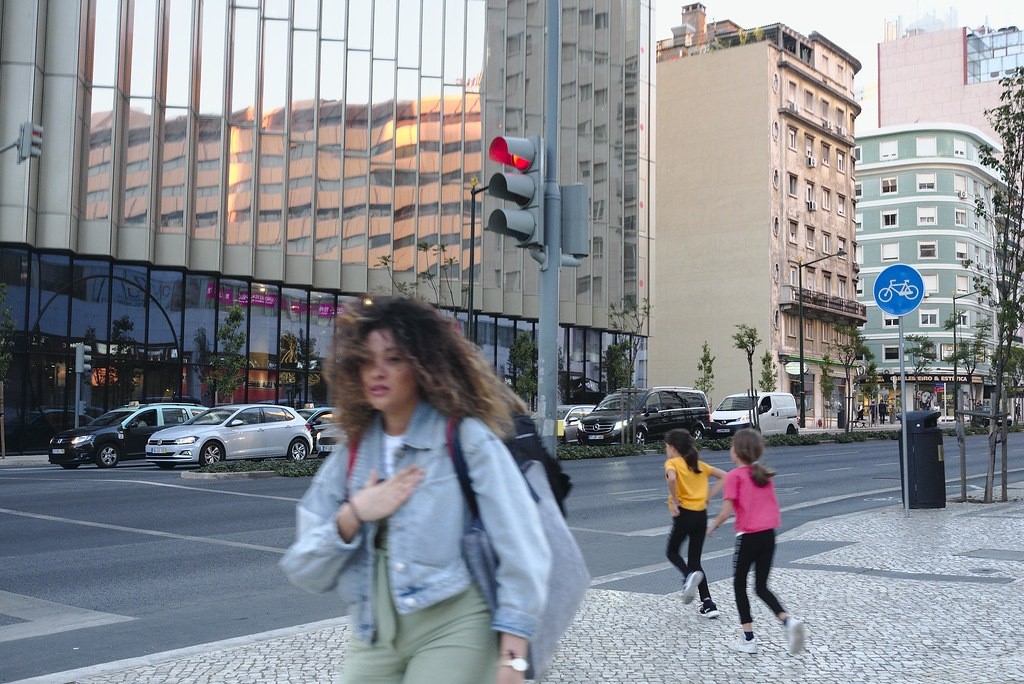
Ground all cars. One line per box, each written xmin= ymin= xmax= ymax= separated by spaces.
xmin=531 ymin=405 xmax=610 ymax=446
xmin=144 ymin=405 xmax=315 ymax=469
xmin=970 ymin=406 xmax=1013 ymax=427
xmin=315 ymin=427 xmax=349 ymax=456
xmin=48 ymin=403 xmax=220 ymax=470
xmin=296 ymin=407 xmax=341 ymax=431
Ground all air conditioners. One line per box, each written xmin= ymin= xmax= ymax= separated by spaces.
xmin=959 ymin=191 xmax=968 ymax=199
xmin=824 ymin=121 xmax=833 ymax=131
xmin=838 ymin=127 xmax=846 ymax=137
xmin=808 ymin=157 xmax=816 ymax=167
xmin=979 ymin=263 xmax=984 ymax=270
xmin=791 ymin=102 xmax=801 ymax=113
xmin=809 ymin=201 xmax=817 ymax=211
xmin=989 ymin=267 xmax=994 ymax=274
xmin=978 ymin=297 xmax=983 ymax=303
xmin=963 ymin=259 xmax=968 ymax=266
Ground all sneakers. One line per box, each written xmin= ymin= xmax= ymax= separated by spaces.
xmin=682 ymin=570 xmax=705 ymax=605
xmin=699 ymin=602 xmax=720 ymax=618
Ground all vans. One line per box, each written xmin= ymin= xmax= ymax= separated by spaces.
xmin=709 ymin=390 xmax=799 ymax=437
xmin=575 ymin=386 xmax=711 ymax=445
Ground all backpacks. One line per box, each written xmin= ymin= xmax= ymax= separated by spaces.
xmin=493 ymin=411 xmax=575 ymax=520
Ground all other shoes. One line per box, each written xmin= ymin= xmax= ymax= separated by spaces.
xmin=783 ymin=617 xmax=805 ymax=654
xmin=728 ymin=634 xmax=758 ymax=654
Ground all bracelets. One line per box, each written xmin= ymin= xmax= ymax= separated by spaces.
xmin=347 ymin=500 xmax=365 ymax=524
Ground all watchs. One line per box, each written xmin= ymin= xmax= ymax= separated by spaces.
xmin=498 ymin=658 xmax=531 ymax=671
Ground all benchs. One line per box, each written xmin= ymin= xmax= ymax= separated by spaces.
xmin=850 ymin=420 xmax=868 ymax=428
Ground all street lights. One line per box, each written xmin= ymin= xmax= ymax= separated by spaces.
xmin=797 ymin=250 xmax=849 ymax=427
xmin=952 ymin=290 xmax=982 ymax=419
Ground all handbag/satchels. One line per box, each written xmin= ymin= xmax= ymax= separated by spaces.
xmin=442 ymin=408 xmax=592 ymax=678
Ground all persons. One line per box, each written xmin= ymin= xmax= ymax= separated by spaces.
xmin=869 ymin=400 xmax=876 ymax=424
xmin=1016 ymin=404 xmax=1020 ymax=420
xmin=857 ymin=402 xmax=864 ymax=420
xmin=879 ymin=399 xmax=886 ymax=424
xmin=664 ymin=428 xmax=728 ymax=618
xmin=705 ymin=428 xmax=804 ymax=654
xmin=276 ymin=299 xmax=551 ymax=684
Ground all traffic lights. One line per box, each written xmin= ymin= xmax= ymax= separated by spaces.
xmin=483 ymin=134 xmax=545 ymax=247
xmin=16 ymin=122 xmax=44 ymax=164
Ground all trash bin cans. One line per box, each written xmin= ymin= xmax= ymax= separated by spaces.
xmin=837 ymin=409 xmax=846 ymax=429
xmin=897 ymin=412 xmax=947 ymax=508
